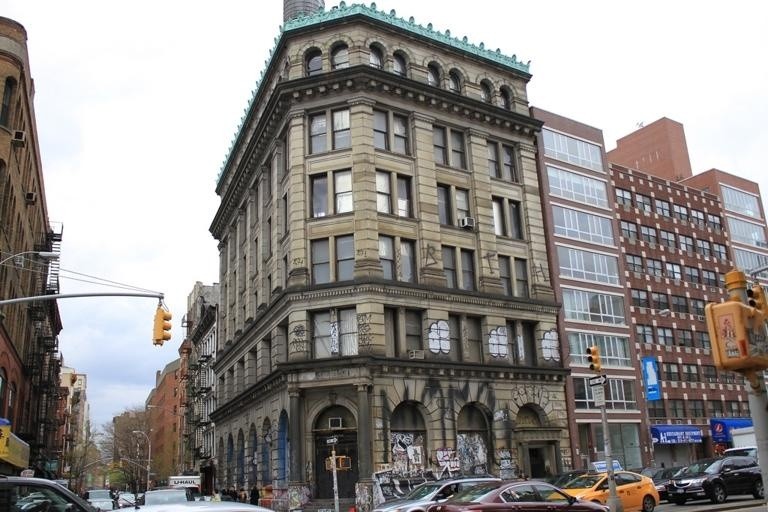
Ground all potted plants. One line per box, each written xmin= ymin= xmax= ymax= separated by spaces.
xmin=192 ymin=492 xmax=204 ymax=501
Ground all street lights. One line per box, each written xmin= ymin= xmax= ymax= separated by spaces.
xmin=147 ymin=404 xmax=216 ymax=503
xmin=119 ymin=430 xmax=152 ymax=491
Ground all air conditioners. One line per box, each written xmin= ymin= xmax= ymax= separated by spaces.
xmin=407 ymin=350 xmax=426 ymax=360
xmin=458 ymin=216 xmax=476 ymax=229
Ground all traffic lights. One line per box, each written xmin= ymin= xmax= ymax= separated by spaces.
xmin=586 ymin=346 xmax=600 ymax=371
xmin=746 ymin=284 xmax=768 ymax=321
xmin=336 ymin=457 xmax=351 ymax=468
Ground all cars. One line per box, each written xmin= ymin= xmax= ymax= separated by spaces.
xmin=0 ymin=475 xmax=275 ymax=512
xmin=371 ymin=475 xmax=611 ymax=512
xmin=664 ymin=446 xmax=764 ymax=505
xmin=543 ymin=467 xmax=687 ymax=512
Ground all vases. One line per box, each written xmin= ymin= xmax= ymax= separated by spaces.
xmin=204 ymin=495 xmax=212 ymax=501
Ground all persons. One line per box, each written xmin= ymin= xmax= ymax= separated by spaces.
xmin=228 ymin=486 xmax=237 ymax=502
xmin=249 ymin=485 xmax=259 ymax=507
xmin=238 ymin=488 xmax=247 ymax=503
xmin=221 ymin=484 xmax=229 ymax=496
